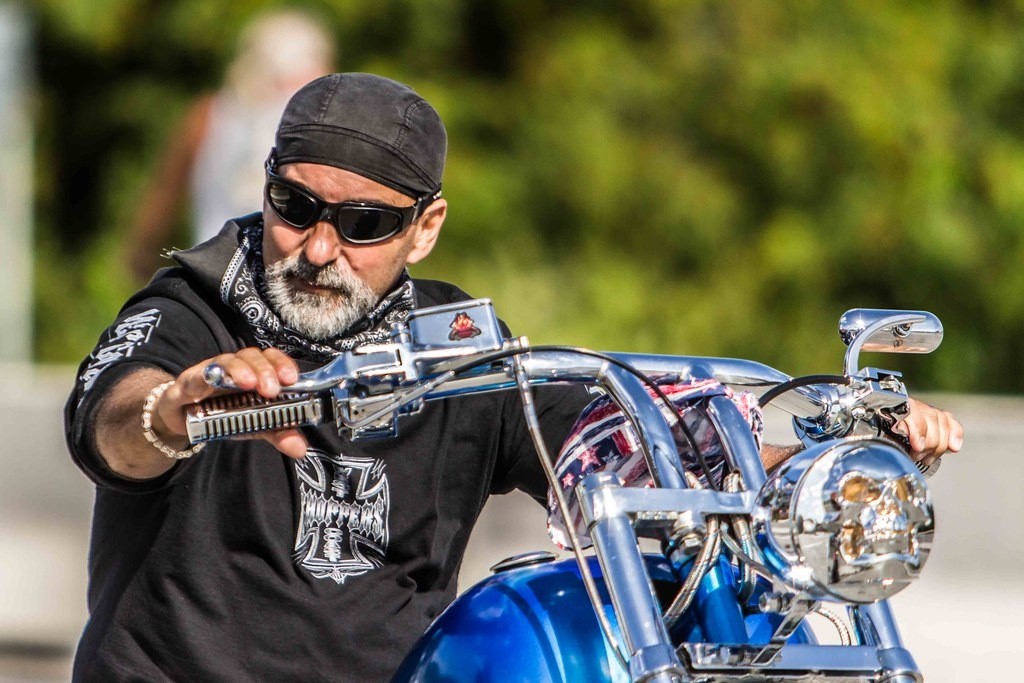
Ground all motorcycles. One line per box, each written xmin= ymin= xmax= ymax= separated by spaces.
xmin=183 ymin=299 xmax=957 ymax=683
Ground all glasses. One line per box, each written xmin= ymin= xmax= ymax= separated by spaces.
xmin=262 ymin=147 xmax=441 ymax=245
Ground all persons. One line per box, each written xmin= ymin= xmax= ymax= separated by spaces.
xmin=192 ymin=14 xmax=336 ymax=246
xmin=62 ymin=72 xmax=964 ymax=683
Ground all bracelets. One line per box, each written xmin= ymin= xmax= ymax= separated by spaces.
xmin=139 ymin=377 xmax=207 ymax=461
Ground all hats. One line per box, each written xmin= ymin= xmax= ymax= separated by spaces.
xmin=264 ymin=72 xmax=448 ymax=201
